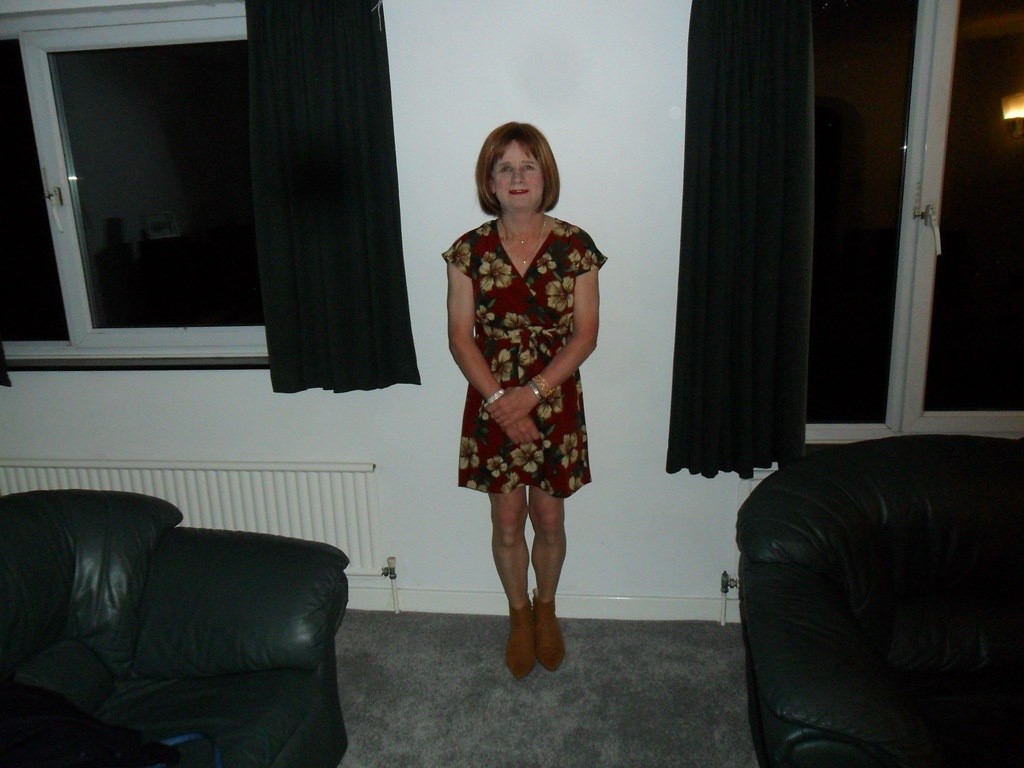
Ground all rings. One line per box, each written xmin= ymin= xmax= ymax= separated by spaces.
xmin=494 ymin=412 xmax=498 ymax=418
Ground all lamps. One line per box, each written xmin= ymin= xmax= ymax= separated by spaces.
xmin=1003 ymin=90 xmax=1024 ymax=138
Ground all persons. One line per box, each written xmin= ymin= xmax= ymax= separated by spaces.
xmin=441 ymin=122 xmax=608 ymax=680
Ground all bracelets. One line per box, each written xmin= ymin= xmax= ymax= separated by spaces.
xmin=487 ymin=389 xmax=505 ymax=405
xmin=528 ymin=374 xmax=553 ymax=404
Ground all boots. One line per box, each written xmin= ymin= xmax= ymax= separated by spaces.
xmin=532 ymin=588 xmax=564 ymax=671
xmin=505 ymin=594 xmax=535 ymax=678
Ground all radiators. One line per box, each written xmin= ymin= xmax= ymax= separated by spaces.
xmin=0 ymin=457 xmax=385 ymax=576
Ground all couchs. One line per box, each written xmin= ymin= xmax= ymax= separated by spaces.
xmin=735 ymin=435 xmax=1024 ymax=768
xmin=1 ymin=490 xmax=350 ymax=768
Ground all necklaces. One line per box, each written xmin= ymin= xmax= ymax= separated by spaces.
xmin=498 ymin=215 xmax=546 ymax=264
